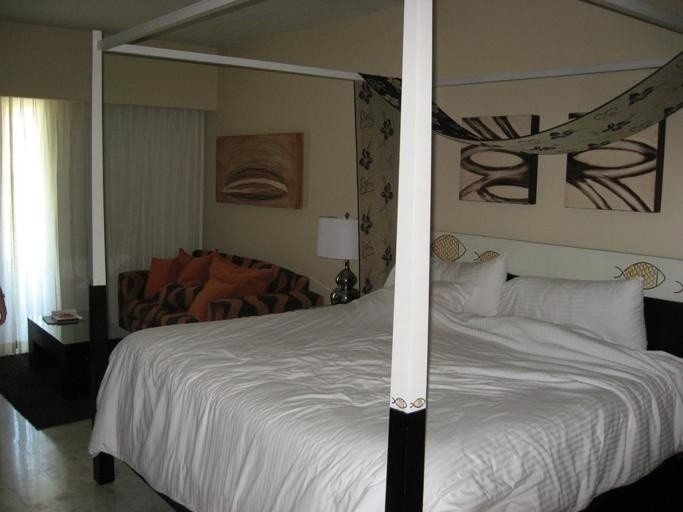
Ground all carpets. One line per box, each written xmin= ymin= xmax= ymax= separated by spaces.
xmin=0 ymin=351 xmax=96 ymax=431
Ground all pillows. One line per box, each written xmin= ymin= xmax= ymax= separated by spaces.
xmin=384 ymin=253 xmax=651 ymax=353
xmin=142 ymin=248 xmax=279 ymax=323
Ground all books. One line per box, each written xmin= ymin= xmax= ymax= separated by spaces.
xmin=42 ymin=309 xmax=83 ymax=325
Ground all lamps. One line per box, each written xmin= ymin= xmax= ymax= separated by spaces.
xmin=312 ymin=213 xmax=363 ymax=304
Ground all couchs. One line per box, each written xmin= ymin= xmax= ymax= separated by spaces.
xmin=119 ymin=247 xmax=322 ymax=333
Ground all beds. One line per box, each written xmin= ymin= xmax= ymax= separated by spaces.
xmin=89 ymin=231 xmax=681 ymax=509
